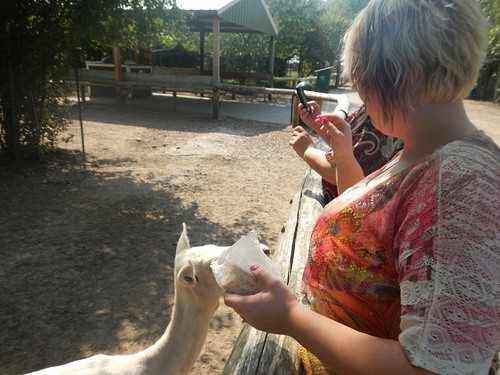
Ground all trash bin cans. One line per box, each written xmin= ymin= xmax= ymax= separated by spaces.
xmin=313 ymin=65 xmax=335 ymax=93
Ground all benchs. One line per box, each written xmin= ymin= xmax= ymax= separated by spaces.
xmin=202 ymin=71 xmax=274 ymax=100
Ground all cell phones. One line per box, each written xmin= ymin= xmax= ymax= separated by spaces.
xmin=296 ymin=86 xmax=312 ymax=111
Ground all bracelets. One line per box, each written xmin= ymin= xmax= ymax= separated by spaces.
xmin=303 ymin=149 xmax=309 ymax=160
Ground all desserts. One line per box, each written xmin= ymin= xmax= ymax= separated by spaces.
xmin=210 ymin=230 xmax=282 ymax=297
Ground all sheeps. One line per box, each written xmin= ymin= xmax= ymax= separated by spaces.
xmin=19 ymin=220 xmax=269 ymax=375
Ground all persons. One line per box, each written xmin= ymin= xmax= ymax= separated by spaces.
xmin=289 ymin=99 xmax=405 ymax=201
xmin=223 ymin=0 xmax=500 ymax=375
